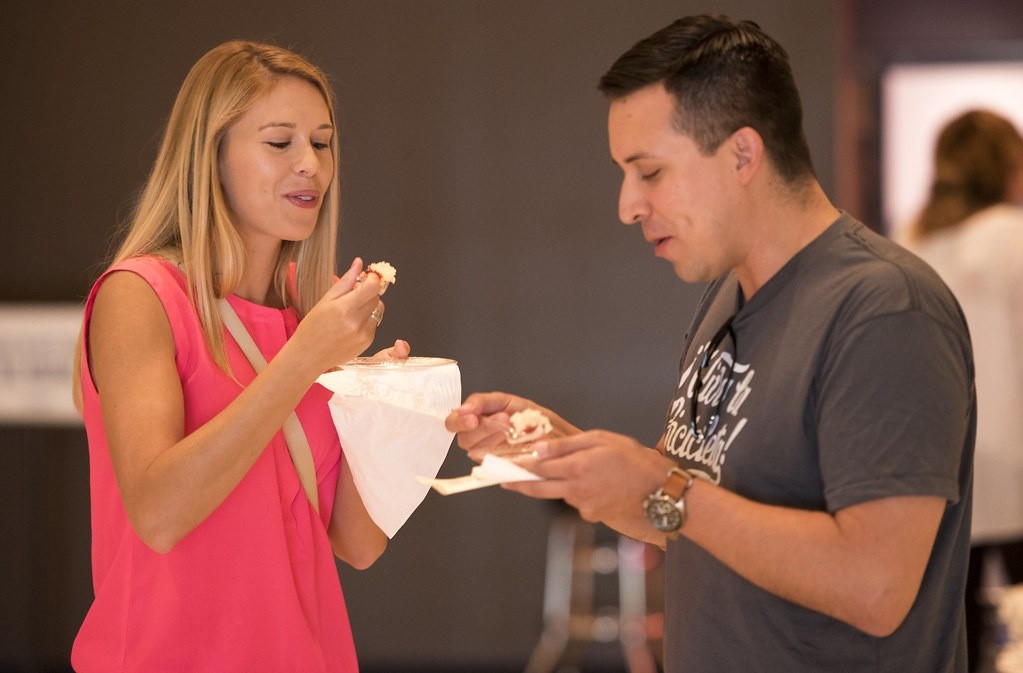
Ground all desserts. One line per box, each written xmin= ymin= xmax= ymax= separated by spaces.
xmin=366 ymin=261 xmax=397 ymax=284
xmin=509 ymin=407 xmax=553 ymax=440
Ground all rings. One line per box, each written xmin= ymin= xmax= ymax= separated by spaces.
xmin=371 ymin=308 xmax=384 ymax=325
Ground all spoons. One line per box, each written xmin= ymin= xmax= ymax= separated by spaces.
xmin=473 ymin=413 xmax=543 ymax=443
xmin=355 ymin=270 xmax=394 ymax=297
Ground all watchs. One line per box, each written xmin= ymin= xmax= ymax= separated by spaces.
xmin=641 ymin=464 xmax=694 ymax=533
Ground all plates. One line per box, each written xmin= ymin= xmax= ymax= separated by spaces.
xmin=466 ymin=440 xmax=536 ymax=466
xmin=334 ymin=357 xmax=458 ymax=378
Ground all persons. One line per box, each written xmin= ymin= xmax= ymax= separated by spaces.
xmin=896 ymin=107 xmax=1023 ymax=588
xmin=441 ymin=12 xmax=979 ymax=670
xmin=71 ymin=36 xmax=414 ymax=671
